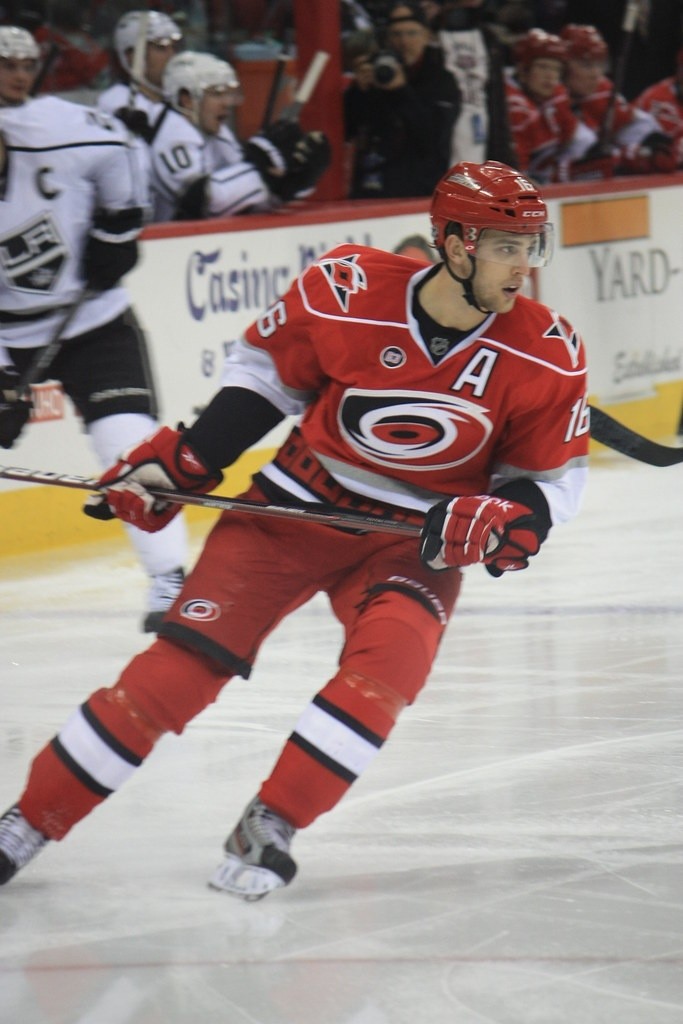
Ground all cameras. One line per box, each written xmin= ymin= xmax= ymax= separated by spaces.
xmin=365 ymin=50 xmax=398 ymax=85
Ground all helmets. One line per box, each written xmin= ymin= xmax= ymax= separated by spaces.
xmin=430 ymin=160 xmax=554 ymax=255
xmin=560 ymin=23 xmax=607 ymax=61
xmin=113 ymin=10 xmax=184 ymax=70
xmin=162 ymin=50 xmax=241 ymax=104
xmin=511 ymin=28 xmax=573 ymax=65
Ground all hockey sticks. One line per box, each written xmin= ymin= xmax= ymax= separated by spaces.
xmin=598 ymin=1 xmax=640 ymax=144
xmin=0 ymin=465 xmax=422 ymax=537
xmin=16 ymin=286 xmax=92 ymax=400
xmin=259 ymin=30 xmax=291 ymax=127
xmin=29 ymin=43 xmax=59 ymax=97
xmin=285 ymin=50 xmax=329 ymax=122
xmin=588 ymin=405 xmax=683 ymax=467
xmin=128 ymin=83 xmax=137 ymax=116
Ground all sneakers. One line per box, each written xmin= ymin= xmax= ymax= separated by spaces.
xmin=208 ymin=794 xmax=299 ymax=902
xmin=141 ymin=567 xmax=185 ymax=633
xmin=0 ymin=802 xmax=52 ymax=885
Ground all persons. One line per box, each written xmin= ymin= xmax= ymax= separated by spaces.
xmin=96 ymin=0 xmax=683 ymax=225
xmin=0 ymin=160 xmax=588 ymax=903
xmin=0 ymin=27 xmax=187 ymax=634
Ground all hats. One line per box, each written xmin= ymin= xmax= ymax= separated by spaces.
xmin=386 ymin=2 xmax=430 ymax=28
xmin=0 ymin=25 xmax=42 ymax=61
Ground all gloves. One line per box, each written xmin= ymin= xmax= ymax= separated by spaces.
xmin=420 ymin=493 xmax=540 ymax=573
xmin=244 ymin=116 xmax=330 ymax=201
xmin=82 ymin=426 xmax=223 ymax=532
xmin=0 ymin=366 xmax=33 ymax=449
xmin=79 ymin=208 xmax=144 ymax=291
